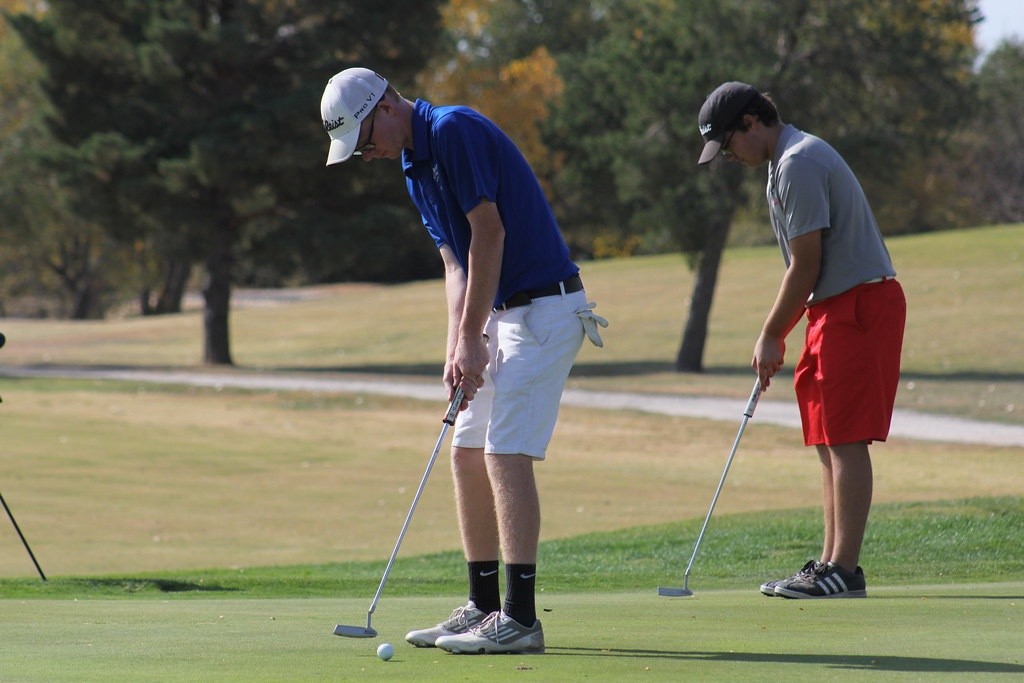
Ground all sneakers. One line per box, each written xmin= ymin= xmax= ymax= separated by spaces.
xmin=776 ymin=563 xmax=867 ymax=598
xmin=435 ymin=611 xmax=546 ymax=654
xmin=760 ymin=558 xmax=817 ymax=596
xmin=405 ymin=598 xmax=489 ymax=647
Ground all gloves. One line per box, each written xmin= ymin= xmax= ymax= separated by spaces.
xmin=575 ymin=302 xmax=609 ymax=348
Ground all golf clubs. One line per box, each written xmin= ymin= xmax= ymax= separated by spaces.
xmin=656 ymin=376 xmax=762 ymax=597
xmin=332 ymin=333 xmax=490 ymax=639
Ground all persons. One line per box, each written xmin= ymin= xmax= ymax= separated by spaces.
xmin=319 ymin=66 xmax=608 ymax=653
xmin=697 ymin=82 xmax=906 ymax=599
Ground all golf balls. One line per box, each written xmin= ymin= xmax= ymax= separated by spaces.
xmin=377 ymin=643 xmax=395 ymax=661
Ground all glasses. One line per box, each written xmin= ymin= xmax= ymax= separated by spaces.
xmin=720 ymin=116 xmax=745 ymax=159
xmin=353 ymin=94 xmax=386 ymax=156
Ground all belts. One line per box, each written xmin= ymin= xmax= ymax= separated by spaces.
xmin=490 ymin=273 xmax=584 ymax=313
xmin=865 ymin=273 xmax=894 ymax=284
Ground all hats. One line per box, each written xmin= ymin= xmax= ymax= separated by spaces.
xmin=319 ymin=65 xmax=389 ymax=167
xmin=697 ymin=82 xmax=758 ymax=165
